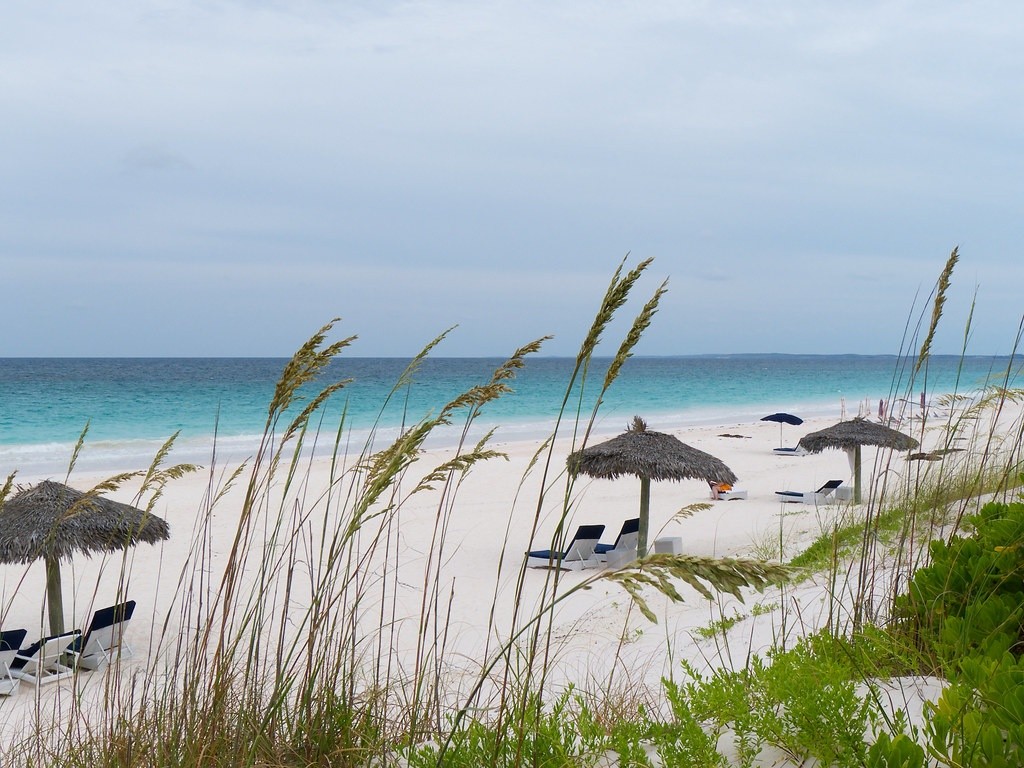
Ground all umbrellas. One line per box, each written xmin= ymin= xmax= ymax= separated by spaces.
xmin=0 ymin=481 xmax=171 ymax=636
xmin=920 ymin=391 xmax=925 ymax=408
xmin=566 ymin=416 xmax=739 ymax=569
xmin=759 ymin=412 xmax=803 ymax=448
xmin=878 ymin=399 xmax=883 ymax=419
xmin=799 ymin=416 xmax=920 ymax=504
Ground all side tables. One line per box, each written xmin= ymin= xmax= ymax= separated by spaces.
xmin=802 ymin=492 xmax=826 ymax=506
xmin=653 ymin=536 xmax=682 ymax=556
xmin=834 ymin=486 xmax=853 ymax=500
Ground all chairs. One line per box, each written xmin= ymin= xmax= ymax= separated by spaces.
xmin=525 ymin=525 xmax=605 ymax=568
xmin=0 ymin=629 xmax=27 ymax=696
xmin=592 ymin=518 xmax=639 ymax=561
xmin=67 ymin=600 xmax=136 ymax=670
xmin=708 ymin=481 xmax=748 ymax=501
xmin=5 ymin=629 xmax=81 ymax=685
xmin=775 ymin=480 xmax=843 ymax=505
xmin=773 ymin=438 xmax=806 ymax=456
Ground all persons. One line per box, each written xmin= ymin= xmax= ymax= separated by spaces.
xmin=708 ymin=480 xmax=731 ymax=500
